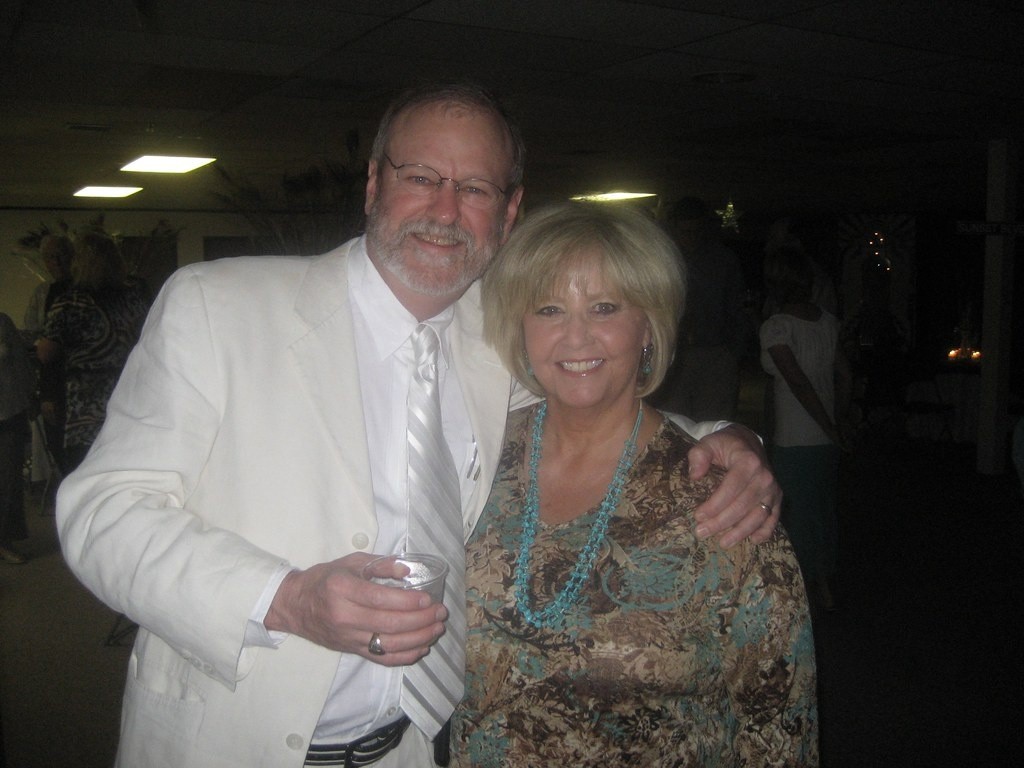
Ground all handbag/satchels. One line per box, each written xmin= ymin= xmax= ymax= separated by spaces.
xmin=37 ymin=361 xmax=74 ymax=405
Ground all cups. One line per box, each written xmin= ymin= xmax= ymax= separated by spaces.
xmin=362 ymin=554 xmax=448 ymax=606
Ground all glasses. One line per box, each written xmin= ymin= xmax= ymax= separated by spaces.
xmin=382 ymin=151 xmax=506 ymax=210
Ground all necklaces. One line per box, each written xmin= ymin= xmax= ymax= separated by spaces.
xmin=514 ymin=398 xmax=645 ymax=628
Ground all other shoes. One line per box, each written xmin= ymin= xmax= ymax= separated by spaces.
xmin=0 ymin=539 xmax=26 ymax=564
xmin=812 ymin=585 xmax=837 ymax=611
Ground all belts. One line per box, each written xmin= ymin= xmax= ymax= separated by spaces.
xmin=304 ymin=714 xmax=412 ymax=768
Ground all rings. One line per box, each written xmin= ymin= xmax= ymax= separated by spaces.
xmin=759 ymin=501 xmax=771 ymax=514
xmin=368 ymin=632 xmax=385 ymax=655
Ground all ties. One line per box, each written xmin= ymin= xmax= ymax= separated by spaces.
xmin=399 ymin=323 xmax=467 ymax=740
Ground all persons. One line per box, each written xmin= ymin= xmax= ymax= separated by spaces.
xmin=657 ymin=197 xmax=855 ymax=620
xmin=0 ymin=229 xmax=148 ymax=565
xmin=55 ymin=80 xmax=784 ymax=768
xmin=446 ymin=196 xmax=820 ymax=767
